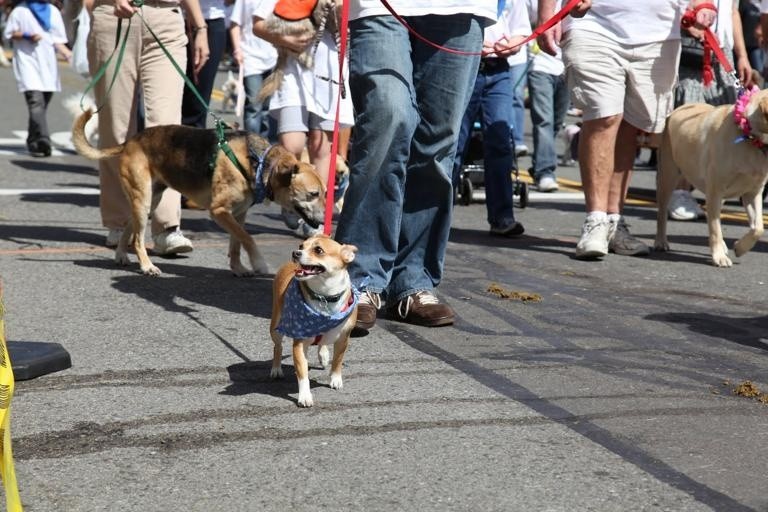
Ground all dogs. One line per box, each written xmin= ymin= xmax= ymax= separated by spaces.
xmin=655 ymin=88 xmax=768 ymax=268
xmin=270 ymin=233 xmax=359 ymax=407
xmin=558 ymin=121 xmax=582 ymax=166
xmin=222 ymin=70 xmax=240 ymax=111
xmin=72 ymin=109 xmax=327 ymax=281
xmin=300 ymin=143 xmax=350 ymax=219
xmin=255 ymin=0 xmax=342 ymax=106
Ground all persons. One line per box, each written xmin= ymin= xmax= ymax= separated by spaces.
xmin=2 ymin=2 xmax=766 ymax=262
xmin=252 ymin=0 xmax=503 ymax=334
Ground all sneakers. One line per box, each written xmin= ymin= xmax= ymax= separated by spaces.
xmin=353 ymin=291 xmax=381 ymax=329
xmin=386 ymin=290 xmax=455 ymax=326
xmin=29 ymin=137 xmax=304 ymax=255
xmin=535 ymin=125 xmax=706 ymax=256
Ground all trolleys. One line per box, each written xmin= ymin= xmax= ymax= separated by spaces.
xmin=444 ymin=137 xmax=532 ymax=217
